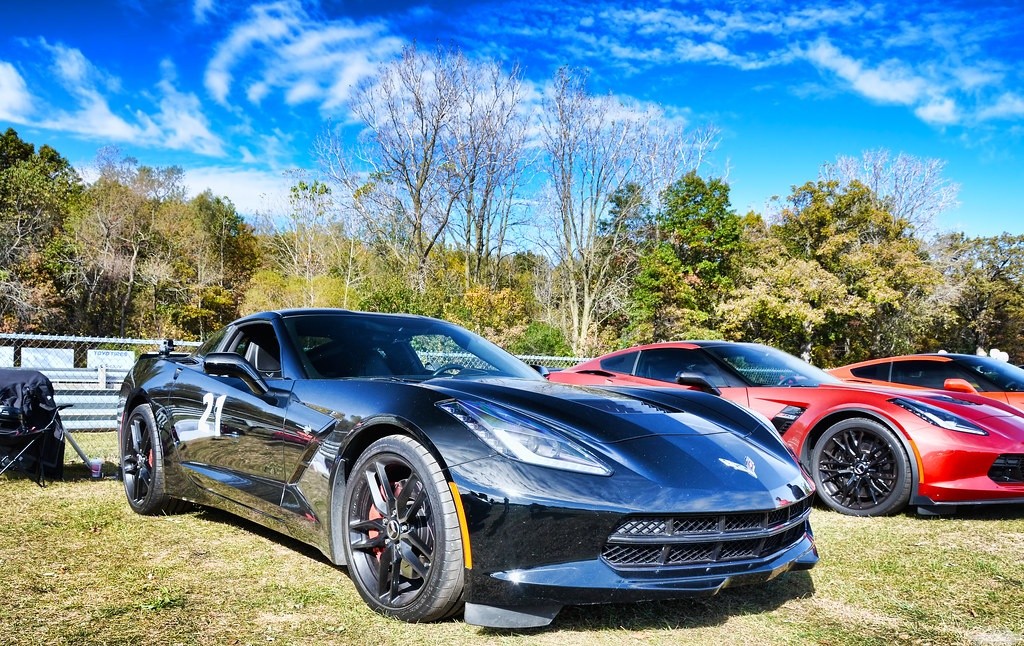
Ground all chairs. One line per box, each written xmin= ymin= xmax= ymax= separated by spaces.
xmin=688 ymin=353 xmax=726 ymax=387
xmin=646 ymin=351 xmax=681 ymax=383
xmin=247 ymin=330 xmax=281 ymax=378
xmin=330 ymin=331 xmax=393 ymax=379
xmin=0 ymin=368 xmax=75 ymax=488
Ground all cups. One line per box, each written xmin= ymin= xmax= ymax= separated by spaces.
xmin=91 ymin=460 xmax=102 ymax=478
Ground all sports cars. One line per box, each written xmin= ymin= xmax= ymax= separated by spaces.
xmin=116 ymin=306 xmax=821 ymax=629
xmin=777 ymin=353 xmax=1024 ymax=415
xmin=546 ymin=339 xmax=1024 ymax=518
